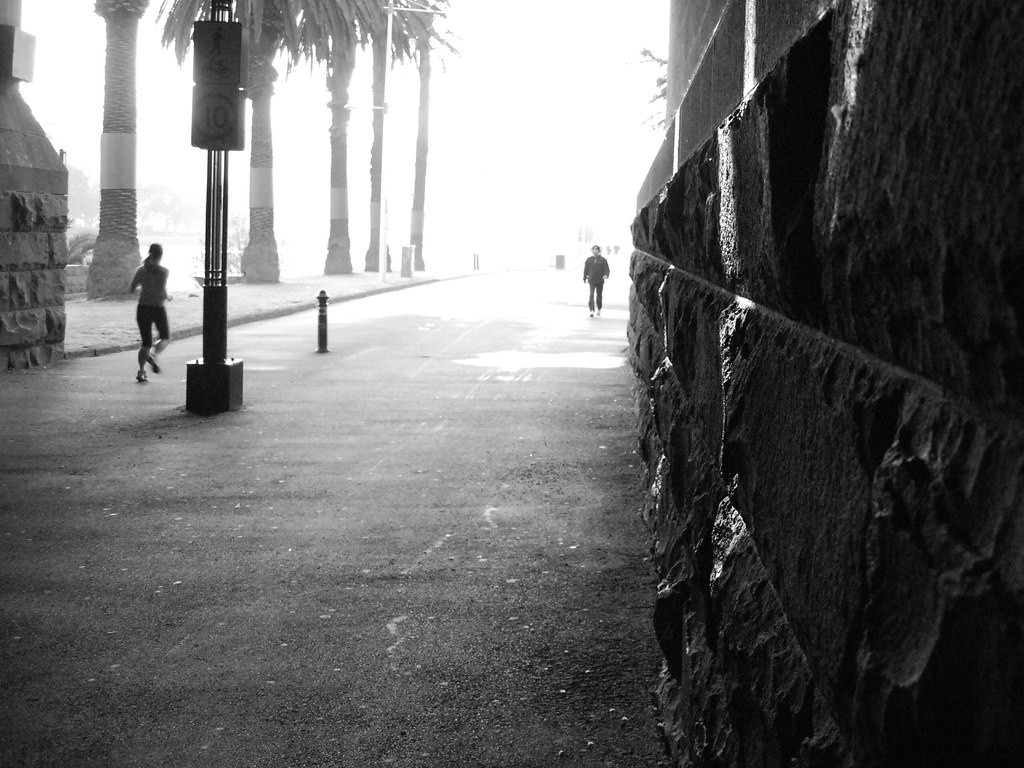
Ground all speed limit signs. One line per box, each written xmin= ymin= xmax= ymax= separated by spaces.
xmin=194 ymin=93 xmax=237 ymax=138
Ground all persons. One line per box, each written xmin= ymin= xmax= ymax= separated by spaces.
xmin=584 ymin=245 xmax=610 ymax=316
xmin=129 ymin=243 xmax=173 ymax=381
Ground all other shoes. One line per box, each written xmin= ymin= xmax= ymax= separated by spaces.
xmin=589 ymin=310 xmax=594 ymax=318
xmin=596 ymin=308 xmax=601 ymax=315
xmin=147 ymin=355 xmax=161 ymax=373
xmin=136 ymin=370 xmax=149 ymax=381
